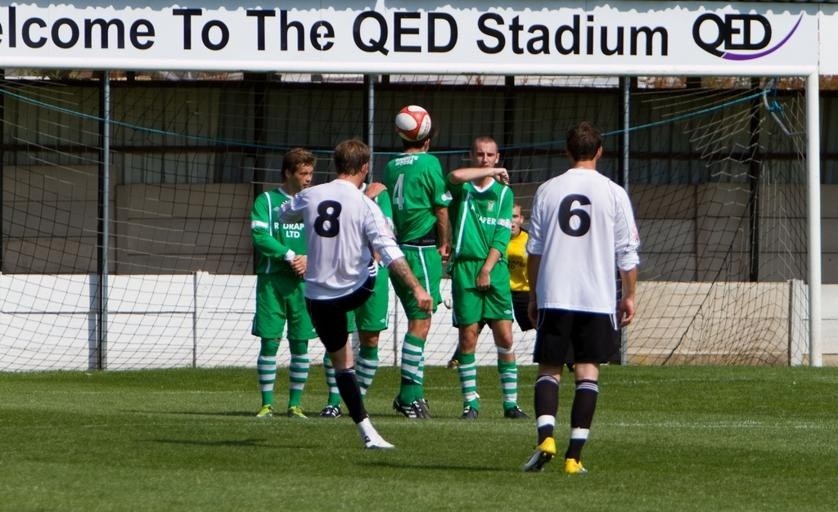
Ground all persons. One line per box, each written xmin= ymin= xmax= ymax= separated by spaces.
xmin=383 ymin=128 xmax=453 ymax=418
xmin=320 ymin=169 xmax=398 ymax=419
xmin=250 ymin=148 xmax=319 ymax=418
xmin=448 ymin=203 xmax=577 ymax=371
xmin=523 ymin=122 xmax=640 ymax=473
xmin=445 ymin=134 xmax=531 ymax=421
xmin=277 ymin=140 xmax=433 ymax=448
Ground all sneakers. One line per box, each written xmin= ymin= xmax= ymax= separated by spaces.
xmin=504 ymin=406 xmax=531 ymax=419
xmin=287 ymin=406 xmax=308 ymax=418
xmin=447 ymin=360 xmax=460 ymax=369
xmin=523 ymin=437 xmax=556 ymax=472
xmin=364 ymin=436 xmax=395 ymax=448
xmin=567 ymin=363 xmax=576 ymax=372
xmin=256 ymin=403 xmax=273 ymax=418
xmin=319 ymin=405 xmax=343 ymax=419
xmin=564 ymin=458 xmax=588 ymax=474
xmin=462 ymin=405 xmax=478 ymax=419
xmin=393 ymin=395 xmax=425 ymax=419
xmin=416 ymin=398 xmax=433 ymax=419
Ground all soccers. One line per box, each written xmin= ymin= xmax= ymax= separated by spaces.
xmin=394 ymin=104 xmax=432 ymax=142
